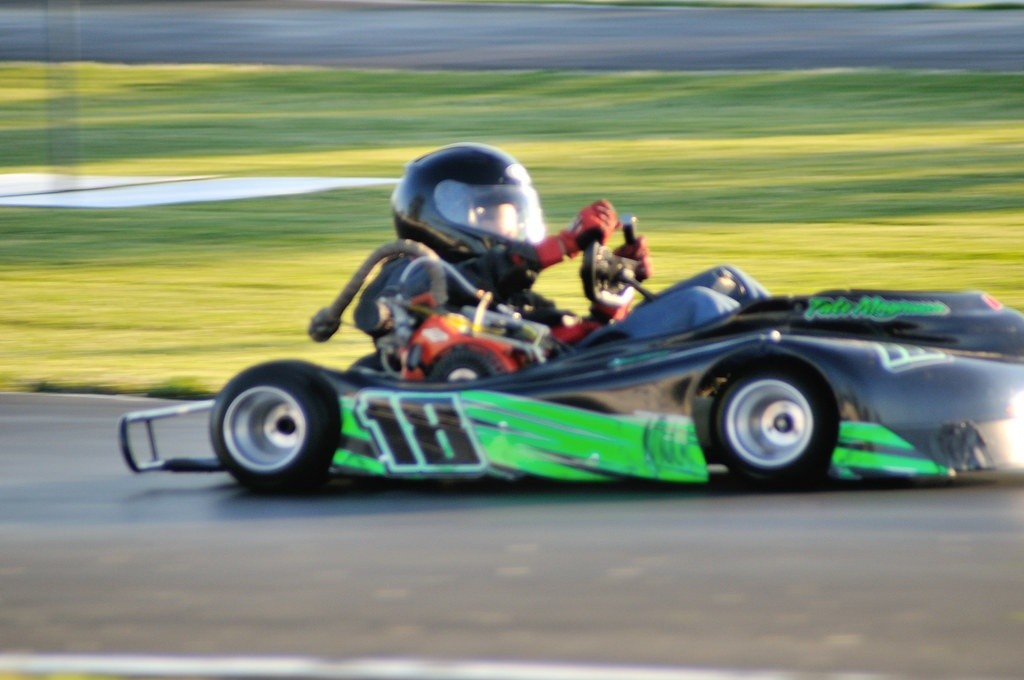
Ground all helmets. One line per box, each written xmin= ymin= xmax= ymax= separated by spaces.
xmin=391 ymin=142 xmax=545 ymax=289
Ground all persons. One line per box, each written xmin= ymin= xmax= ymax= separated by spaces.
xmin=351 ymin=140 xmax=776 ymax=385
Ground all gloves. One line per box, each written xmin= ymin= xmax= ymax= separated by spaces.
xmin=559 ymin=199 xmax=623 ymax=259
xmin=613 ymin=236 xmax=652 ymax=283
